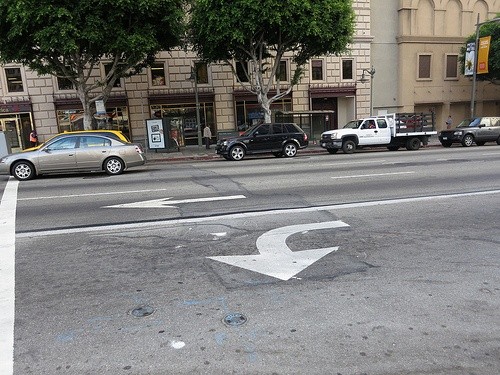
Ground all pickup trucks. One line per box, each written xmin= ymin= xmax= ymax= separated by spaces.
xmin=319 ymin=111 xmax=438 ymax=154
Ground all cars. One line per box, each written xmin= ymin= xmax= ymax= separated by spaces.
xmin=0 ymin=133 xmax=147 ymax=181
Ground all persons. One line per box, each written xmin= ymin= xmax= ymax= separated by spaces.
xmin=446 ymin=115 xmax=452 ymax=129
xmin=203 ymin=123 xmax=212 ymax=149
xmin=368 ymin=122 xmax=374 ymax=129
xmin=30 ymin=130 xmax=37 ymax=147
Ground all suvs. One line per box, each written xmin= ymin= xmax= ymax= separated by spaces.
xmin=212 ymin=122 xmax=309 ymax=161
xmin=436 ymin=116 xmax=500 ymax=148
xmin=16 ymin=129 xmax=132 ymax=153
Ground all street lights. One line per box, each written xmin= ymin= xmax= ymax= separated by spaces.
xmin=357 ymin=64 xmax=375 ymax=116
xmin=185 ymin=66 xmax=208 ymax=160
